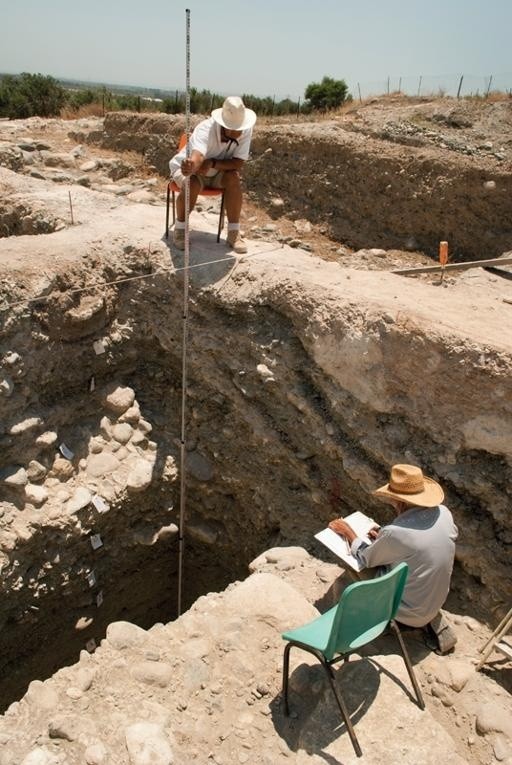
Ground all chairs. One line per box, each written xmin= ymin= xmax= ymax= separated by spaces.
xmin=282 ymin=564 xmax=427 ymax=757
xmin=165 ymin=129 xmax=228 ymax=247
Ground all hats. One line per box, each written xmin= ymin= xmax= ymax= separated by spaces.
xmin=371 ymin=462 xmax=446 ymax=508
xmin=211 ymin=96 xmax=257 ymax=131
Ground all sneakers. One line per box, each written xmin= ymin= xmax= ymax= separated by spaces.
xmin=173 ymin=227 xmax=192 ymax=250
xmin=225 ymin=230 xmax=248 ymax=253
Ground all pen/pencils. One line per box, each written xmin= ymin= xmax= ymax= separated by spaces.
xmin=368 ymin=527 xmax=381 ymax=534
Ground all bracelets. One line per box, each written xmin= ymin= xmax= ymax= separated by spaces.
xmin=211 ymin=158 xmax=217 ymax=167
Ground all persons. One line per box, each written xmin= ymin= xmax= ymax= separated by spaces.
xmin=328 ymin=463 xmax=459 ymax=634
xmin=168 ymin=96 xmax=257 ymax=255
